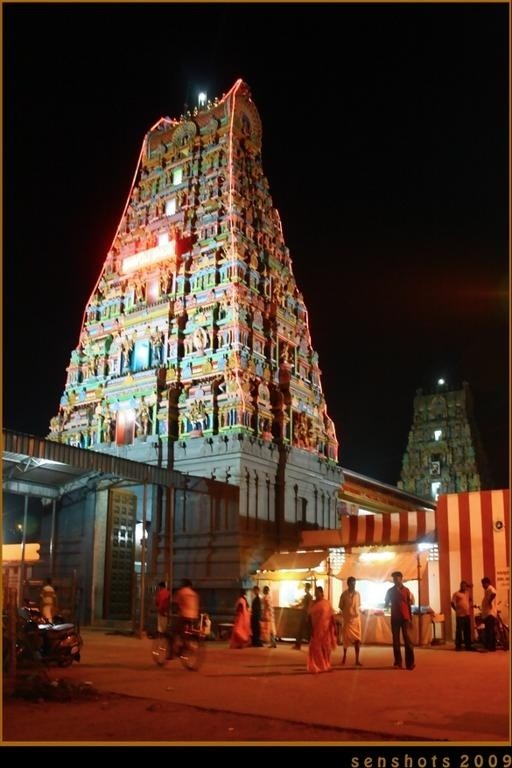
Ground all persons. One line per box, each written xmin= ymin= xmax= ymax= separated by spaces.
xmin=258 ymin=586 xmax=277 ymax=643
xmin=481 ymin=578 xmax=498 ymax=652
xmin=155 ymin=582 xmax=171 ymax=634
xmin=451 ymin=581 xmax=475 ymax=651
xmin=384 ymin=572 xmax=416 ymax=670
xmin=339 ymin=577 xmax=362 ymax=666
xmin=39 ymin=577 xmax=55 ymax=624
xmin=307 ymin=587 xmax=338 ymax=673
xmin=292 ymin=583 xmax=312 ymax=650
xmin=165 ymin=579 xmax=200 ymax=662
xmin=248 ymin=586 xmax=262 ymax=647
xmin=230 ymin=589 xmax=253 ymax=649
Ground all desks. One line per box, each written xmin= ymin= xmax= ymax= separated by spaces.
xmin=336 ymin=611 xmax=436 ymax=646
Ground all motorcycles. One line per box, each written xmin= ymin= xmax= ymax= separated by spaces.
xmin=15 ymin=607 xmax=80 ymax=667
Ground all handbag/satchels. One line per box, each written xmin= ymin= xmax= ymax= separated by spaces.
xmin=258 ymin=621 xmax=270 ymax=642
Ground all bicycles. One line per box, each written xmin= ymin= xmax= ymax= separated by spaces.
xmin=152 ymin=611 xmax=205 ymax=672
